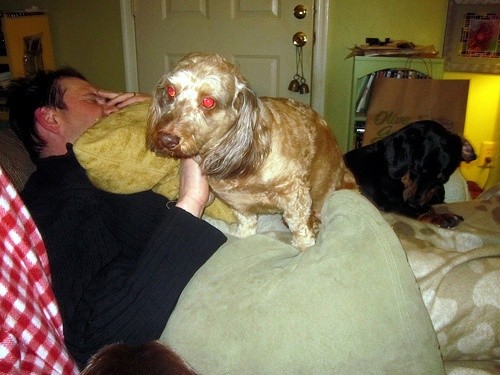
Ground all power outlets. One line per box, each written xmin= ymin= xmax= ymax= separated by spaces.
xmin=478 ymin=142 xmax=499 ymax=168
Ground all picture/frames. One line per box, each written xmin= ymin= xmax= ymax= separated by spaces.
xmin=442 ymin=0 xmax=500 ymax=75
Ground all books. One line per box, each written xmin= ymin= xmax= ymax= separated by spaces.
xmin=356 ymin=67 xmax=430 ymax=114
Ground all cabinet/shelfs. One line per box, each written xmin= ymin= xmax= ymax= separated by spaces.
xmin=346 ymin=49 xmax=445 ymax=154
xmin=0 ymin=14 xmax=56 ymax=122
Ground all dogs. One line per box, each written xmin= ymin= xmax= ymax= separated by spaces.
xmin=343 ymin=120 xmax=476 ymax=228
xmin=143 ymin=51 xmax=358 ymax=252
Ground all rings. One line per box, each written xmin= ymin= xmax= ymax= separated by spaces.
xmin=132 ymin=91 xmax=136 ymax=97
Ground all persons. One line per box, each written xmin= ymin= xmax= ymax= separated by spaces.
xmin=4 ymin=63 xmax=228 ymax=370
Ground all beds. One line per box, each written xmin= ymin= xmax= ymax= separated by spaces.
xmin=433 ymin=196 xmax=499 ymax=234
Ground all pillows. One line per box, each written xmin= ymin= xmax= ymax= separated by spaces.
xmin=0 ymin=98 xmax=243 ymax=375
